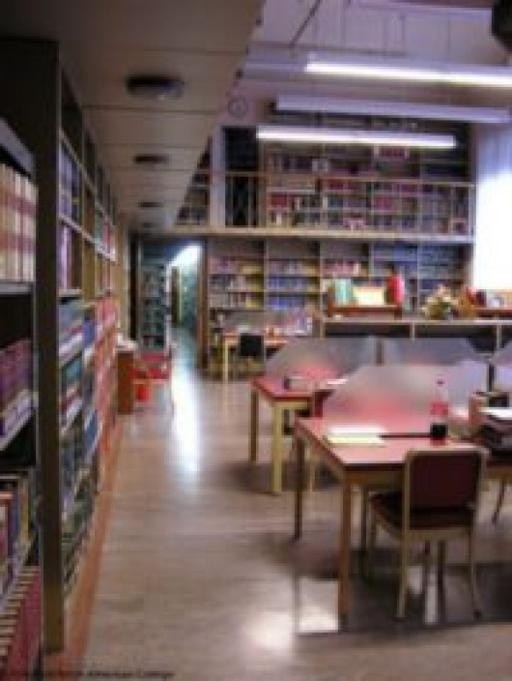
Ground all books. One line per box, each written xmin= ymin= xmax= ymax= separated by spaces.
xmin=141 ymin=262 xmax=178 ymax=359
xmin=323 ymin=434 xmax=387 ymax=448
xmin=1 ymin=118 xmax=128 ymax=681
xmin=208 ymin=127 xmax=476 ymax=376
xmin=328 ymin=425 xmax=390 ymax=436
xmin=465 ymin=386 xmax=512 ymax=452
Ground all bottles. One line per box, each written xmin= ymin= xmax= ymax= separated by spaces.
xmin=429 ymin=377 xmax=449 ymax=447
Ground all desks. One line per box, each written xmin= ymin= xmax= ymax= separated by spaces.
xmin=249 ymin=373 xmax=337 ymax=497
xmin=288 ymin=416 xmax=511 ymax=635
